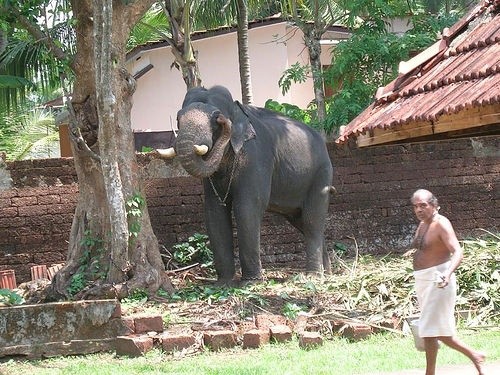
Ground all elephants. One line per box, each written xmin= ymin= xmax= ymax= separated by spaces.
xmin=155 ymin=85 xmax=337 ymax=286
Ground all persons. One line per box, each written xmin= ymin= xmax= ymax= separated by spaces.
xmin=410 ymin=189 xmax=486 ymax=375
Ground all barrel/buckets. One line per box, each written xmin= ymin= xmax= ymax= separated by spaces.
xmin=412 ymin=319 xmax=441 ymax=352
